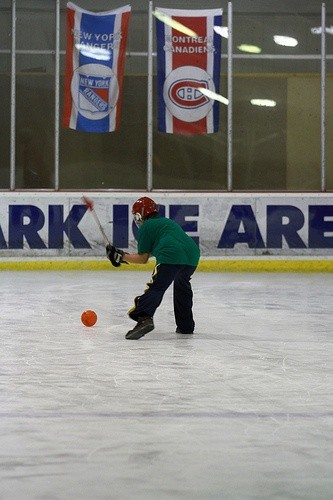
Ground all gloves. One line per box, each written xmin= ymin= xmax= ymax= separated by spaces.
xmin=105 ymin=245 xmax=129 ymax=267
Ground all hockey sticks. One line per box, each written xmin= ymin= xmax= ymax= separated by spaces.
xmin=81 ymin=196 xmax=111 ymax=246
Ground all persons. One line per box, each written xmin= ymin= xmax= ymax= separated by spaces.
xmin=104 ymin=196 xmax=200 ymax=339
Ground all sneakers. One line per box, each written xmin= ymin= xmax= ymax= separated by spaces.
xmin=125 ymin=318 xmax=154 ymax=340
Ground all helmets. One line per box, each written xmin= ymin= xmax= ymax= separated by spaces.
xmin=132 ymin=197 xmax=158 ymax=229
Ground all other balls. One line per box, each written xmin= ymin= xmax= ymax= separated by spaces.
xmin=81 ymin=310 xmax=98 ymax=326
